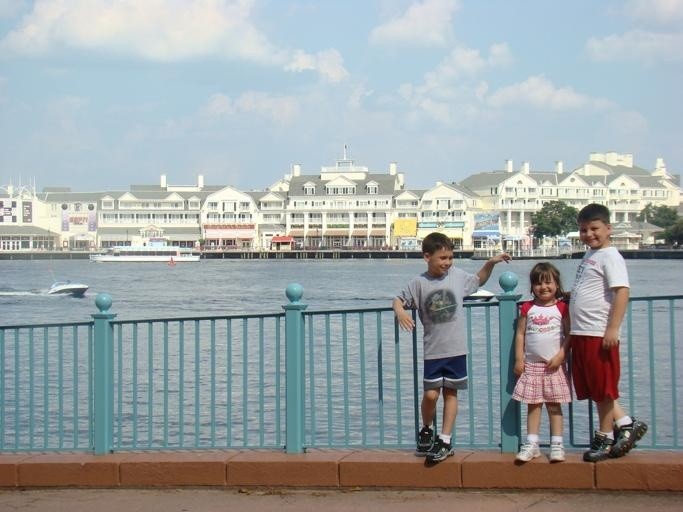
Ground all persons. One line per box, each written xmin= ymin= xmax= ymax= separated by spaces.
xmin=510 ymin=263 xmax=571 ymax=462
xmin=393 ymin=233 xmax=513 ymax=462
xmin=560 ymin=204 xmax=647 ymax=462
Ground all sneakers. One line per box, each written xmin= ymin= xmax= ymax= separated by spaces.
xmin=425 ymin=434 xmax=455 ymax=463
xmin=583 ymin=430 xmax=616 ymax=462
xmin=515 ymin=440 xmax=540 ymax=461
xmin=608 ymin=416 xmax=647 ymax=457
xmin=548 ymin=442 xmax=565 ymax=461
xmin=416 ymin=426 xmax=435 ymax=452
